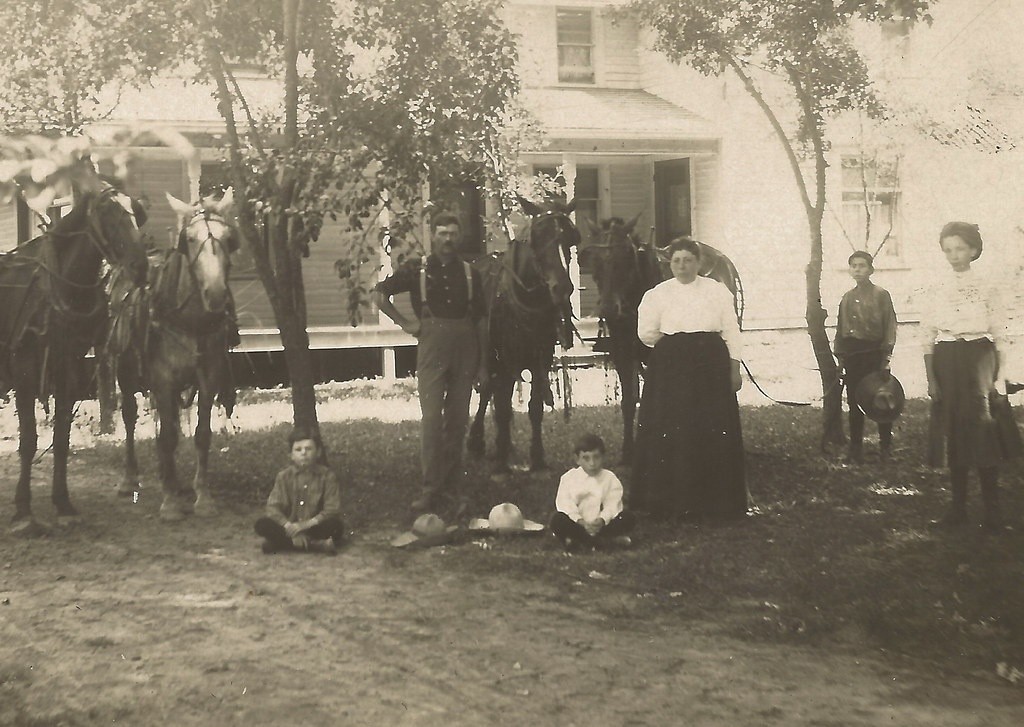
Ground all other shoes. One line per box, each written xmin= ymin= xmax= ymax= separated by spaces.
xmin=312 ymin=537 xmax=336 ymax=555
xmin=608 ymin=535 xmax=633 ymax=550
xmin=882 ymin=444 xmax=897 ymax=463
xmin=263 ymin=539 xmax=290 ymax=553
xmin=929 ymin=509 xmax=967 ymax=526
xmin=413 ymin=491 xmax=441 ymax=510
xmin=841 ymin=456 xmax=864 ymax=465
xmin=986 ymin=520 xmax=1014 ymax=536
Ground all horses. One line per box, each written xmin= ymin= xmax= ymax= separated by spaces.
xmin=460 ymin=190 xmax=744 ymax=485
xmin=0 ymin=176 xmax=238 ymax=539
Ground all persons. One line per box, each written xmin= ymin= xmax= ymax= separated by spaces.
xmin=635 ymin=236 xmax=747 ymax=517
xmin=834 ymin=251 xmax=896 ymax=462
xmin=253 ymin=428 xmax=343 ymax=554
xmin=552 ymin=435 xmax=631 ymax=549
xmin=920 ymin=222 xmax=1005 ymax=530
xmin=374 ymin=215 xmax=484 ymax=510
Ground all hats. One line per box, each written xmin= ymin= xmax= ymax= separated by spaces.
xmin=854 ymin=370 xmax=906 ymax=425
xmin=468 ymin=502 xmax=544 ymax=535
xmin=390 ymin=514 xmax=459 ymax=549
